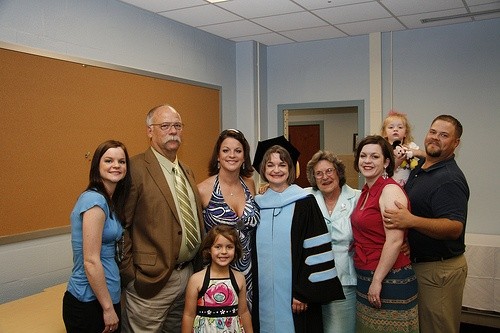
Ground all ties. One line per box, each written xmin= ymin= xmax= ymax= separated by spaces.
xmin=173 ymin=168 xmax=199 ymax=251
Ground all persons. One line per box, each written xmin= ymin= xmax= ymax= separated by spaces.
xmin=62 ymin=140 xmax=133 ymax=333
xmin=119 ymin=105 xmax=470 ymax=333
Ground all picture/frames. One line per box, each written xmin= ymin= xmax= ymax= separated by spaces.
xmin=353 ymin=134 xmax=358 ymax=151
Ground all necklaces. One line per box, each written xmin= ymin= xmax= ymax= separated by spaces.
xmin=230 ymin=193 xmax=232 ymax=196
xmin=328 ymin=211 xmax=332 ymax=215
xmin=116 ymin=236 xmax=124 ymax=262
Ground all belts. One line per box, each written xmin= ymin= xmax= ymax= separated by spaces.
xmin=416 ymin=254 xmax=458 ymax=263
xmin=174 ymin=260 xmax=192 ymax=272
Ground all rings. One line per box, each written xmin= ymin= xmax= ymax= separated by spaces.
xmin=388 ymin=218 xmax=390 ymax=223
xmin=110 ymin=328 xmax=112 ymax=331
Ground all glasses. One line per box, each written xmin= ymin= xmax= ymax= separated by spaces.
xmin=314 ymin=168 xmax=336 ymax=180
xmin=150 ymin=123 xmax=184 ymax=131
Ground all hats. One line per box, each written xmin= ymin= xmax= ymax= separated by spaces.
xmin=252 ymin=135 xmax=300 ymax=179
